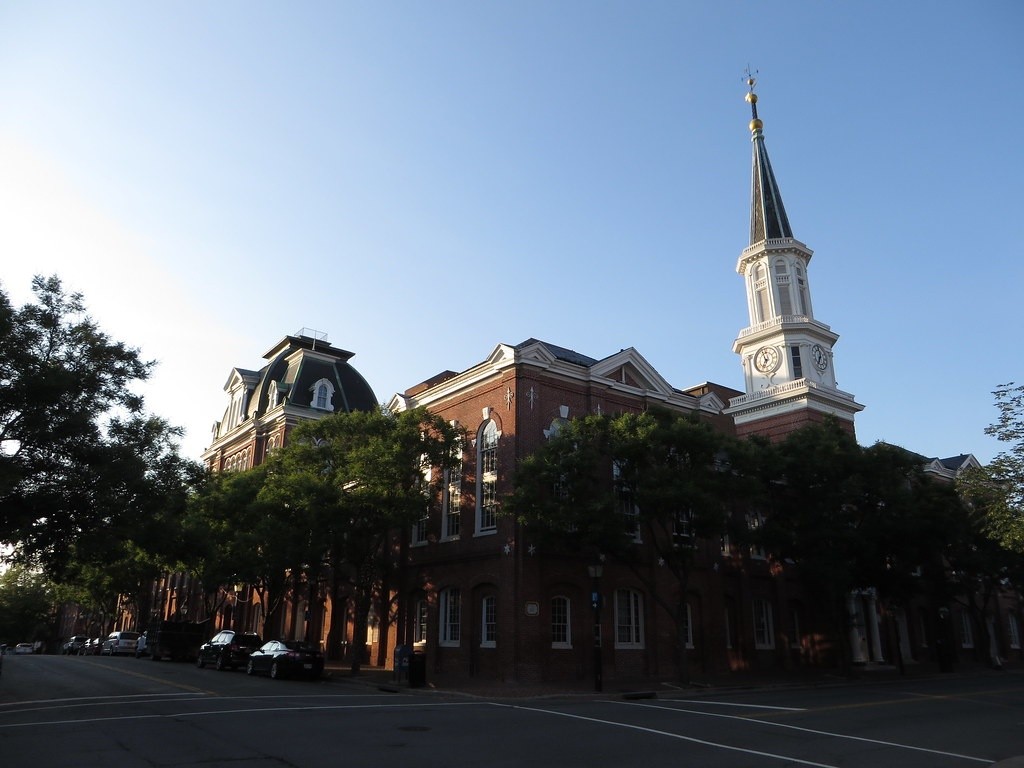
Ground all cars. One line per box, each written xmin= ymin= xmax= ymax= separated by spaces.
xmin=245 ymin=638 xmax=325 ymax=680
xmin=3 ymin=646 xmax=15 ymax=655
xmin=63 ymin=635 xmax=103 ymax=657
xmin=16 ymin=643 xmax=33 ymax=655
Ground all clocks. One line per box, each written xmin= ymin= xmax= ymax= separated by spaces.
xmin=752 ymin=346 xmax=780 ymax=373
xmin=811 ymin=344 xmax=828 ymax=371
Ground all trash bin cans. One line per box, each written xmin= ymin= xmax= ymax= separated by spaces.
xmin=408 ymin=650 xmax=427 ymax=687
xmin=393 ymin=643 xmax=413 ymax=682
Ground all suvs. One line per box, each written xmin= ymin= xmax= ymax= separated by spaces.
xmin=100 ymin=630 xmax=142 ymax=658
xmin=197 ymin=629 xmax=263 ymax=671
